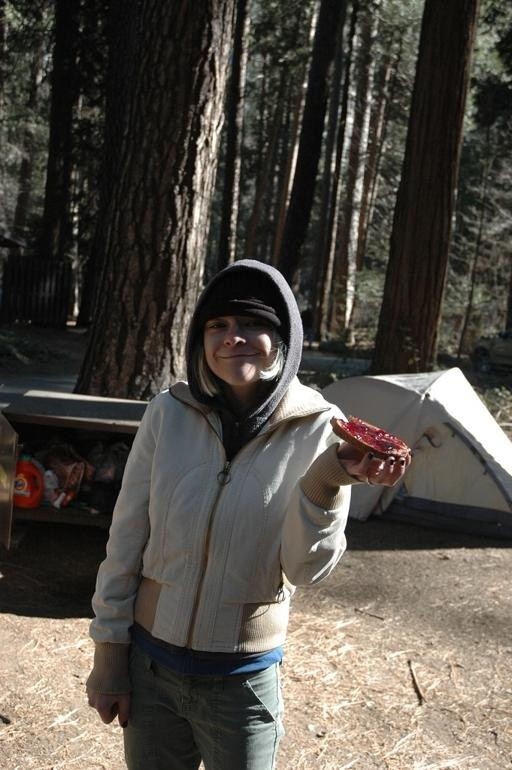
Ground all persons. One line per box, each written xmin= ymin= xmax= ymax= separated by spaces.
xmin=82 ymin=257 xmax=415 ymax=770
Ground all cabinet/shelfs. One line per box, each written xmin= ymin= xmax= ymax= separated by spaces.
xmin=0 ymin=388 xmax=154 ymax=550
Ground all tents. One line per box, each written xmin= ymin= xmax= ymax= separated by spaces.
xmin=317 ymin=366 xmax=511 ymax=525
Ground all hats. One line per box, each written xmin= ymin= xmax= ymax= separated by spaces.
xmin=195 ymin=267 xmax=289 ymax=331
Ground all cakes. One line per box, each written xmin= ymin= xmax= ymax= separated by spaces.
xmin=330 ymin=414 xmax=412 ymax=461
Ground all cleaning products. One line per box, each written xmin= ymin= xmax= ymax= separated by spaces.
xmin=13 ymin=455 xmax=46 ymax=510
